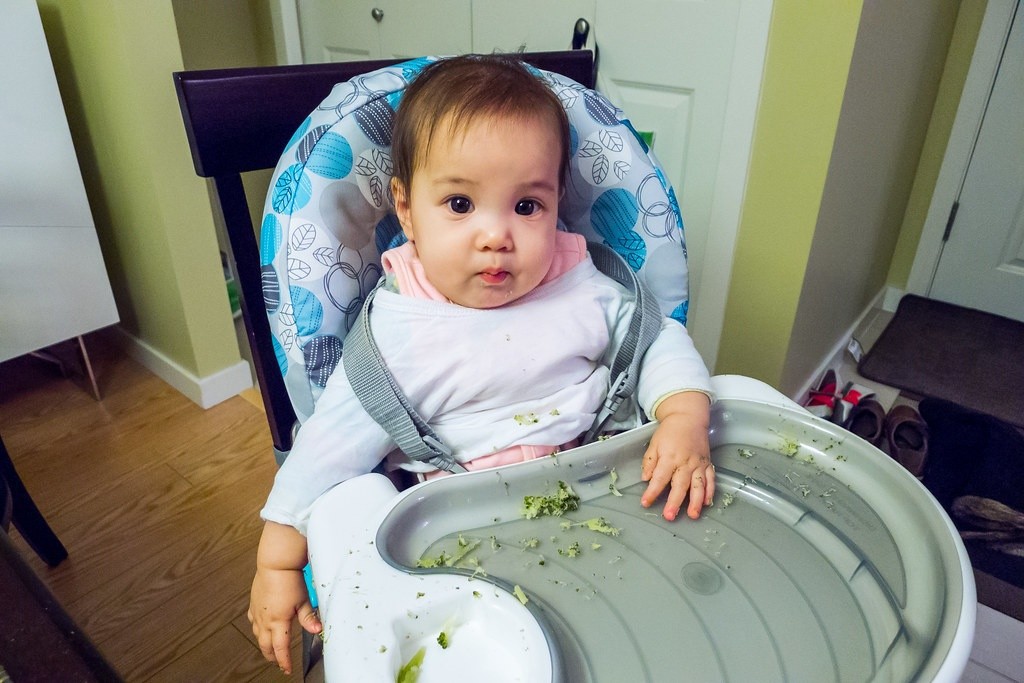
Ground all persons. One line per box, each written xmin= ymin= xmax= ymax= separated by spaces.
xmin=246 ymin=52 xmax=717 ymax=676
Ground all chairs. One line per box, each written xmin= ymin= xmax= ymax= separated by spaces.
xmin=0 ymin=438 xmax=121 ymax=683
xmin=172 ymin=49 xmax=979 ymax=683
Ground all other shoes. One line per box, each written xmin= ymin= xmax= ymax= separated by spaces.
xmin=804 ymin=368 xmax=844 ymax=419
xmin=849 ymin=400 xmax=885 ymax=447
xmin=832 ymin=381 xmax=876 ymax=427
xmin=886 ymin=405 xmax=928 ymax=476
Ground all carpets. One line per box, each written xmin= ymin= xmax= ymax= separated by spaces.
xmin=857 ymin=294 xmax=1024 ymax=425
